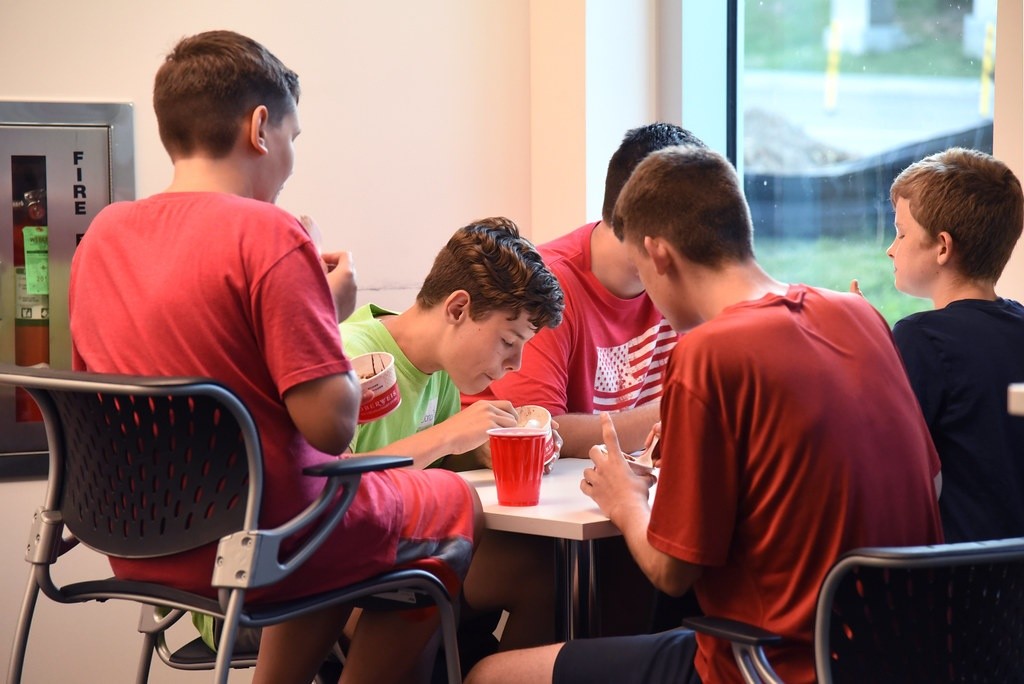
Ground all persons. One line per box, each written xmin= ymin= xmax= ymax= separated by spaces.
xmin=188 ymin=216 xmax=565 ymax=682
xmin=464 ymin=145 xmax=942 ymax=684
xmin=67 ymin=30 xmax=487 ymax=684
xmin=849 ymin=148 xmax=1024 ymax=542
xmin=460 ymin=122 xmax=710 ymax=651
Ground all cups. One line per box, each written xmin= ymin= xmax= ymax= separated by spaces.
xmin=487 ymin=427 xmax=547 ymax=508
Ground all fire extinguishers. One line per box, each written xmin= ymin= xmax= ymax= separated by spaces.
xmin=12 ymin=188 xmax=50 ymax=423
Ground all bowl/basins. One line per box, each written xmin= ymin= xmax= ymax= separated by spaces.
xmin=512 ymin=405 xmax=556 ymax=465
xmin=586 ymin=444 xmax=654 ymax=486
xmin=349 ymin=351 xmax=402 ymax=423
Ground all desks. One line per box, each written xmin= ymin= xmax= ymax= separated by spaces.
xmin=456 ymin=458 xmax=660 ymax=643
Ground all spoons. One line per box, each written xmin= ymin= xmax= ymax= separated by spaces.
xmin=635 ymin=434 xmax=659 ymax=468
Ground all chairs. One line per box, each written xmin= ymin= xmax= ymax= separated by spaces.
xmin=683 ymin=537 xmax=1024 ymax=684
xmin=0 ymin=367 xmax=462 ymax=684
xmin=136 ymin=603 xmax=346 ymax=684
